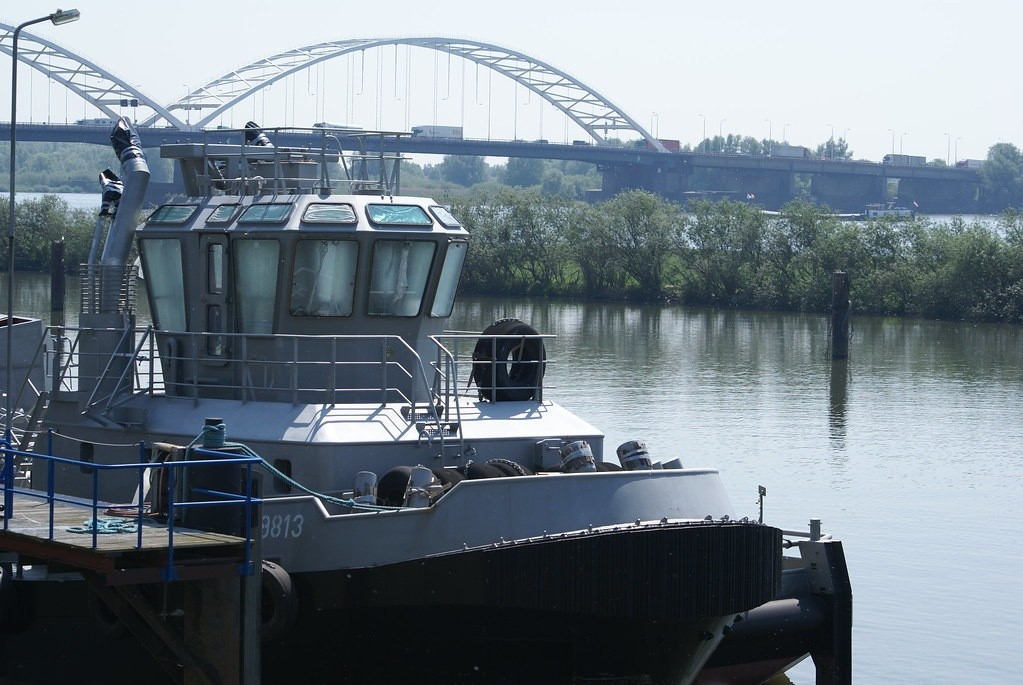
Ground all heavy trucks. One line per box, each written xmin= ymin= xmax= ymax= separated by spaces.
xmin=882 ymin=154 xmax=927 ymax=166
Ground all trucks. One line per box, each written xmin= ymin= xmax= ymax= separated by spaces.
xmin=772 ymin=145 xmax=809 ymax=158
xmin=634 ymin=138 xmax=681 ymax=153
xmin=411 ymin=124 xmax=464 ymax=140
xmin=573 ymin=140 xmax=589 ymax=146
xmin=959 ymin=158 xmax=988 ymax=169
xmin=312 ymin=123 xmax=365 ymax=134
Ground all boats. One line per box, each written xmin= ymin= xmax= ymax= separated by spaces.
xmin=4 ymin=123 xmax=854 ymax=685
xmin=863 ymin=200 xmax=920 ymax=223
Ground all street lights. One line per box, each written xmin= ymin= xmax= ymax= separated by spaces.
xmin=765 ymin=120 xmax=772 ymax=157
xmin=719 ymin=119 xmax=726 ymax=152
xmin=900 ymin=132 xmax=907 ymax=154
xmin=954 ymin=136 xmax=963 ymax=167
xmin=783 ymin=123 xmax=790 ymax=144
xmin=843 ymin=129 xmax=851 ymax=146
xmin=3 ymin=9 xmax=85 ymax=521
xmin=889 ymin=128 xmax=895 ymax=164
xmin=651 ymin=111 xmax=659 ymax=140
xmin=699 ymin=114 xmax=707 ymax=155
xmin=827 ymin=124 xmax=834 ymax=159
xmin=945 ymin=133 xmax=950 ymax=167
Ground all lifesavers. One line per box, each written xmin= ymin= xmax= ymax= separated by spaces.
xmin=471 ymin=317 xmax=547 ymax=402
xmin=86 ymin=576 xmax=137 ymax=642
xmin=375 ymin=461 xmax=534 ymax=511
xmin=239 ymin=558 xmax=300 ymax=649
